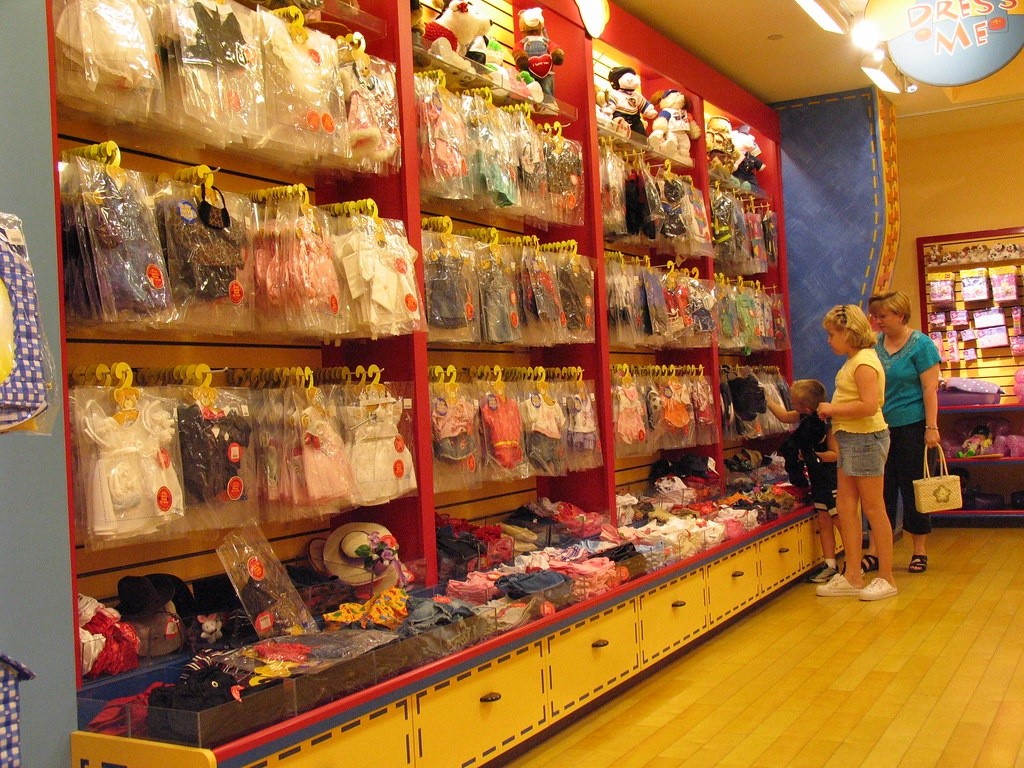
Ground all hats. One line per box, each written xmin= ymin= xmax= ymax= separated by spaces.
xmin=114 ymin=573 xmax=176 ymax=620
xmin=307 ymin=522 xmax=415 ymax=600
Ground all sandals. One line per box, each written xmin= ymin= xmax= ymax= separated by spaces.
xmin=909 ymin=554 xmax=927 ymax=573
xmin=861 ymin=555 xmax=879 ymax=572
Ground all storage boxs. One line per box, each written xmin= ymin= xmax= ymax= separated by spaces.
xmin=928 ymin=275 xmax=1024 ymax=362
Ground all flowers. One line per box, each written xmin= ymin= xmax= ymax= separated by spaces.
xmin=355 ymin=531 xmax=405 ymax=599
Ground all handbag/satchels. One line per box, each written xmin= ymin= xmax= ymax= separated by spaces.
xmin=912 ymin=443 xmax=963 ymax=514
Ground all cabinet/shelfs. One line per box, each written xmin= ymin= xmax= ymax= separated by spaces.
xmin=46 ymin=0 xmax=846 ymax=767
xmin=923 ymin=404 xmax=1024 ymax=527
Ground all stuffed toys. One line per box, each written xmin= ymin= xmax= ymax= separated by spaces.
xmin=647 ymin=89 xmax=701 ymax=159
xmin=705 ymin=115 xmax=767 ymax=191
xmin=512 ymin=7 xmax=565 ymax=113
xmin=596 ymin=66 xmax=658 ymax=138
xmin=410 ymin=0 xmax=511 ymax=103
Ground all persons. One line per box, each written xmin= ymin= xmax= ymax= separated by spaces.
xmin=861 ymin=290 xmax=941 ymax=575
xmin=816 ymin=303 xmax=898 ymax=602
xmin=750 ymin=370 xmax=866 ymax=583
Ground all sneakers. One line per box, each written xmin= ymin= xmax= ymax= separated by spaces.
xmin=816 ymin=573 xmax=865 ymax=596
xmin=840 ymin=561 xmax=846 ymax=575
xmin=860 ymin=577 xmax=897 ymax=600
xmin=806 ymin=561 xmax=839 ymax=582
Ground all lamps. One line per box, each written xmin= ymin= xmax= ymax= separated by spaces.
xmin=859 ymin=55 xmax=904 ymax=94
xmin=794 ymin=0 xmax=857 ymax=35
xmin=907 ymin=83 xmax=919 ymax=92
